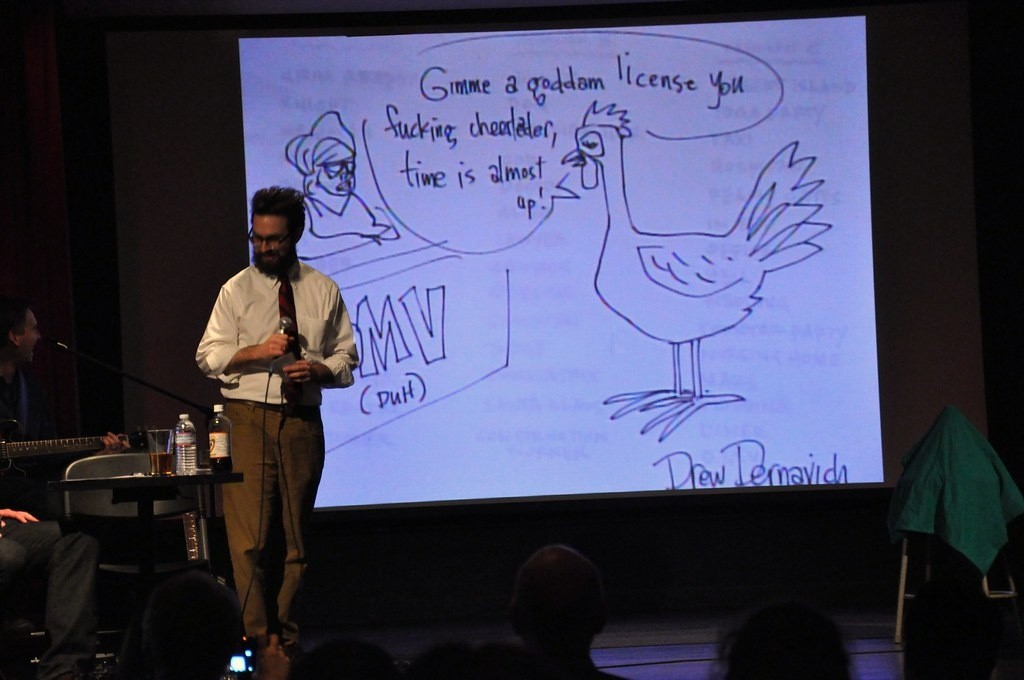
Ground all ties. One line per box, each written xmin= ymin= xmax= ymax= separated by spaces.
xmin=278 ymin=273 xmax=303 ymax=408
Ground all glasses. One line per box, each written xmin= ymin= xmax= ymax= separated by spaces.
xmin=247 ymin=226 xmax=289 ymax=248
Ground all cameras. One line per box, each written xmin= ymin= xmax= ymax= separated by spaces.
xmin=225 ymin=636 xmax=260 ymax=676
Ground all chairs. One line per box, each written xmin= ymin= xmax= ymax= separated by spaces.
xmin=57 ymin=452 xmax=209 ymax=655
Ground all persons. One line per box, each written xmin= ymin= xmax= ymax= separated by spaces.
xmin=903 ymin=577 xmax=1004 ymax=680
xmin=196 ymin=188 xmax=359 ymax=668
xmin=287 ymin=546 xmax=628 ymax=680
xmin=0 ymin=304 xmax=131 ymax=680
xmin=114 ymin=573 xmax=240 ymax=680
xmin=720 ymin=604 xmax=852 ymax=680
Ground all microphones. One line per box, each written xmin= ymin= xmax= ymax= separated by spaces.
xmin=267 ymin=316 xmax=293 ymax=375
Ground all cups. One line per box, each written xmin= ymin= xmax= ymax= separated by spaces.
xmin=147 ymin=429 xmax=175 ymax=475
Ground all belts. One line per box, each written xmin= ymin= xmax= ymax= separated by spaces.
xmin=226 ymin=398 xmax=319 ymax=412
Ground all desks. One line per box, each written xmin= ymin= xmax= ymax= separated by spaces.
xmin=47 ymin=471 xmax=246 ymax=680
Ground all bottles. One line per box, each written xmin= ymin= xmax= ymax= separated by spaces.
xmin=209 ymin=404 xmax=233 ymax=474
xmin=175 ymin=413 xmax=197 ymax=474
